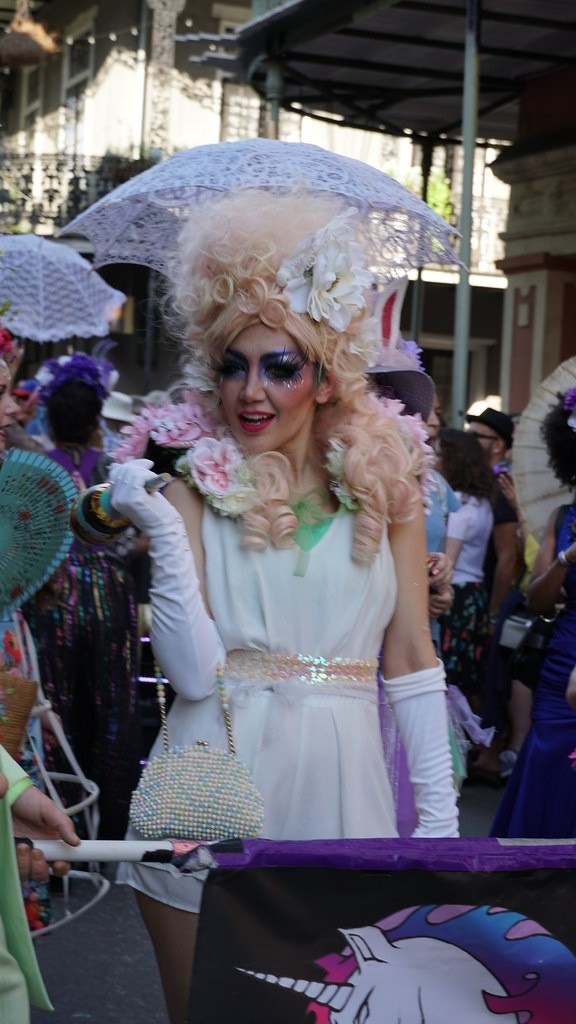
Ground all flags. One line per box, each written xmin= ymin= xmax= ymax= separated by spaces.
xmin=187 ymin=836 xmax=576 ymax=1024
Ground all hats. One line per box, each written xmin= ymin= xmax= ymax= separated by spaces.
xmin=363 ymin=363 xmax=436 ymax=423
xmin=465 ymin=407 xmax=515 ymax=449
xmin=101 ymin=391 xmax=139 ymax=423
xmin=12 ymin=377 xmax=41 ymax=396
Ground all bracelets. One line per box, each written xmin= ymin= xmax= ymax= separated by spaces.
xmin=559 ymin=551 xmax=569 ymax=567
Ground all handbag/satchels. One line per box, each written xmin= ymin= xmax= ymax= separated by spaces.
xmin=129 ymin=659 xmax=266 ymax=840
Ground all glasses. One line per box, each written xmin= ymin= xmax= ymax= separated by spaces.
xmin=467 ymin=430 xmax=497 ymax=440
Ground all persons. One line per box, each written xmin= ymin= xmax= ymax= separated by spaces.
xmin=0 ymin=190 xmax=576 ymax=1024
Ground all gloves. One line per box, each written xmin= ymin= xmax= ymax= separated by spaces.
xmin=101 ymin=457 xmax=225 ymax=702
xmin=380 ymin=655 xmax=461 ymax=837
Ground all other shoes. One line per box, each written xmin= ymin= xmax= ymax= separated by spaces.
xmin=463 ymin=764 xmax=502 ymax=785
xmin=500 ymin=751 xmax=517 ymax=776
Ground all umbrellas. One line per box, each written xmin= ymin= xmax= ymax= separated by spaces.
xmin=0 ymin=447 xmax=80 ymax=621
xmin=511 ymin=356 xmax=576 ymax=547
xmin=54 ymin=137 xmax=470 ymax=284
xmin=0 ymin=231 xmax=127 ymax=342
